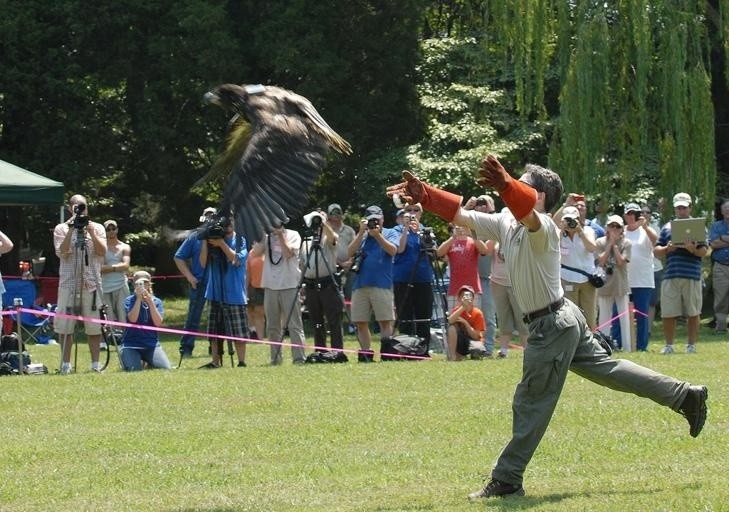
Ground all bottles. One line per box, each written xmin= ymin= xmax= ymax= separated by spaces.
xmin=104 ymin=219 xmax=117 ymax=229
xmin=202 ymin=207 xmax=216 ymax=215
xmin=134 ymin=271 xmax=151 ymax=283
xmin=396 ymin=201 xmax=424 ymax=216
xmin=562 ymin=206 xmax=580 ymax=220
xmin=606 ymin=214 xmax=624 ymax=228
xmin=624 ymin=202 xmax=641 ymax=215
xmin=673 ymin=192 xmax=693 ymax=208
xmin=328 ymin=203 xmax=342 ymax=214
xmin=364 ymin=205 xmax=384 ymax=220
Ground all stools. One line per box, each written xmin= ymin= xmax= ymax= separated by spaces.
xmin=303 ymin=277 xmax=332 ymax=288
xmin=523 ymin=295 xmax=567 ymax=324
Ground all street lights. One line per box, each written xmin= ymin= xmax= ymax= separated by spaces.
xmin=562 ymin=262 xmax=609 ymax=288
xmin=379 ymin=334 xmax=428 ymax=361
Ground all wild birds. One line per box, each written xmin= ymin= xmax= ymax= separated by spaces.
xmin=669 ymin=218 xmax=708 ymax=247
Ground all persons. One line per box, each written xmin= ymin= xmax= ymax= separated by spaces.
xmin=0 ymin=195 xmax=171 ymax=373
xmin=385 ymin=155 xmax=708 ymax=503
xmin=176 ymin=191 xmax=728 ymax=375
xmin=382 ymin=155 xmax=708 ymax=502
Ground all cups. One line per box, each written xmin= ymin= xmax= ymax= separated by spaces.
xmin=105 ymin=228 xmax=116 ymax=231
xmin=330 ymin=211 xmax=341 ymax=216
xmin=406 ymin=208 xmax=420 ymax=213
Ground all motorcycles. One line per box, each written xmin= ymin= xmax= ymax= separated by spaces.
xmin=57 ymin=232 xmax=124 ymax=374
xmin=272 ymin=248 xmax=369 ymax=363
xmin=175 ymin=248 xmax=236 ymax=369
xmin=391 ymin=249 xmax=454 ymax=363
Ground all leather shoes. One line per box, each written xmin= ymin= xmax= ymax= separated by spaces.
xmin=476 ymin=154 xmax=536 ymax=221
xmin=385 ymin=169 xmax=465 ymax=224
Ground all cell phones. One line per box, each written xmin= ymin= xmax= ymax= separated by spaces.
xmin=465 ymin=291 xmax=470 ymax=298
xmin=475 ymin=198 xmax=487 ymax=206
xmin=573 ymin=195 xmax=585 ymax=201
xmin=566 ymin=219 xmax=577 ymax=229
xmin=410 ymin=214 xmax=416 ymax=221
xmin=367 ymin=218 xmax=380 ymax=232
xmin=605 ymin=262 xmax=614 ymax=275
xmin=351 ymin=250 xmax=367 ymax=274
xmin=69 ymin=202 xmax=94 ymax=231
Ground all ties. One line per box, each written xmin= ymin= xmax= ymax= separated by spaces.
xmin=31 ymin=256 xmax=46 ymax=276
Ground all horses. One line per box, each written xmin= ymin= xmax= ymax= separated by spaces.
xmin=634 ymin=210 xmax=641 ymax=221
xmin=138 ymin=280 xmax=144 ymax=294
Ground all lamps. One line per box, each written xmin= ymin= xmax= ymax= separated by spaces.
xmin=1 ymin=279 xmax=57 ymax=345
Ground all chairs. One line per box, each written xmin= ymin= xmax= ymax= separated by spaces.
xmin=660 ymin=319 xmax=729 ymax=355
xmin=681 ymin=385 xmax=708 ymax=438
xmin=60 ymin=338 xmax=309 ymax=369
xmin=467 ymin=475 xmax=525 ymax=502
